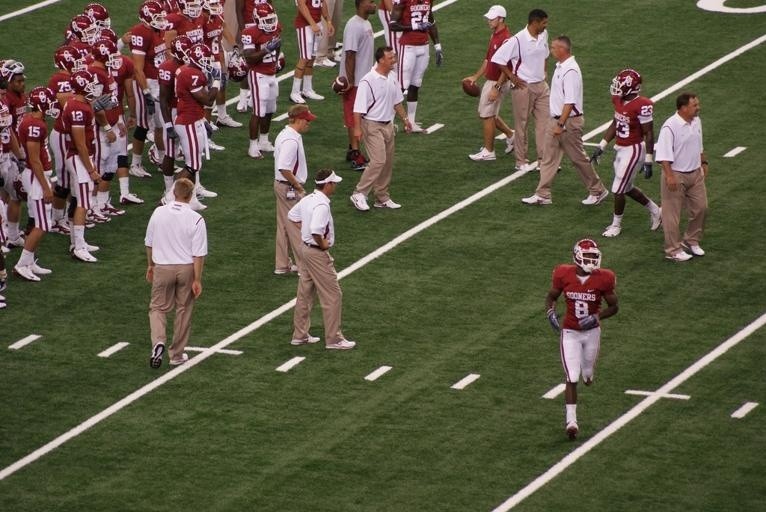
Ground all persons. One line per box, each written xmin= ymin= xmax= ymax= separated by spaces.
xmin=379 ymin=0 xmax=443 ymax=131
xmin=542 ymin=238 xmax=620 ymax=441
xmin=285 ymin=167 xmax=356 ymax=350
xmin=589 ymin=68 xmax=663 ymax=239
xmin=142 ymin=177 xmax=207 ymax=369
xmin=273 ymin=105 xmax=316 ymax=272
xmin=1 ymin=1 xmax=147 ymax=312
xmin=339 ymin=1 xmax=378 ymax=160
xmin=130 ymin=1 xmax=344 ymax=207
xmin=349 ymin=48 xmax=411 ymax=210
xmin=655 ymin=92 xmax=709 ymax=263
xmin=489 ymin=7 xmax=562 ymax=174
xmin=460 ymin=5 xmax=515 ymax=162
xmin=519 ymin=35 xmax=609 ymax=207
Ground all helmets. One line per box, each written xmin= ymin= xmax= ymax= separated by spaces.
xmin=573 ymin=238 xmax=601 ymax=273
xmin=0 ymin=0 xmax=279 ymax=120
xmin=610 ymin=69 xmax=641 ymax=97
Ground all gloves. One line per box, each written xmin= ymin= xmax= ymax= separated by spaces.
xmin=434 ymin=43 xmax=443 ymax=66
xmin=412 ymin=22 xmax=431 ymax=31
xmin=639 ymin=161 xmax=653 ymax=179
xmin=548 ymin=310 xmax=561 ymax=330
xmin=578 ymin=314 xmax=596 ymax=329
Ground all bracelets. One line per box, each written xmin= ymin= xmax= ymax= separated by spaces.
xmin=644 ymin=153 xmax=654 ymax=162
xmin=494 ymin=83 xmax=502 ymax=92
xmin=701 ymin=159 xmax=710 ymax=165
xmin=556 ymin=122 xmax=565 ymax=130
xmin=598 ymin=138 xmax=608 ymax=151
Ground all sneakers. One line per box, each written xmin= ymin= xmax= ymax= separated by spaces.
xmin=582 ymin=372 xmax=593 ymax=384
xmin=169 ymin=353 xmax=189 ymax=365
xmin=326 ymin=339 xmax=355 ymax=349
xmin=150 ymin=342 xmax=165 ymax=368
xmin=346 ymin=146 xmax=402 ymax=211
xmin=248 ymin=138 xmax=275 ymax=159
xmin=566 ymin=422 xmax=579 ymax=439
xmin=291 ymin=335 xmax=320 ymax=345
xmin=405 ymin=121 xmax=423 ymax=133
xmin=602 ymin=205 xmax=705 ymax=262
xmin=289 ymin=88 xmax=324 ymax=104
xmin=1 ymin=136 xmax=224 ymax=281
xmin=468 ymin=130 xmax=609 ymax=205
xmin=275 ymin=261 xmax=297 ymax=274
xmin=314 ymin=56 xmax=336 ymax=68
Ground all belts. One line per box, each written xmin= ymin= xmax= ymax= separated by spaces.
xmin=304 ymin=241 xmax=323 ymax=248
xmin=276 ymin=180 xmax=288 ymax=184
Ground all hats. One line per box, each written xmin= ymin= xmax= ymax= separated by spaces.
xmin=315 ymin=169 xmax=342 ymax=184
xmin=484 ymin=6 xmax=506 ymax=19
xmin=289 ymin=105 xmax=316 ymax=121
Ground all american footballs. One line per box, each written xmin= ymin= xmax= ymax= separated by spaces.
xmin=462 ymin=78 xmax=482 ymax=97
xmin=332 ymin=76 xmax=348 ymax=94
